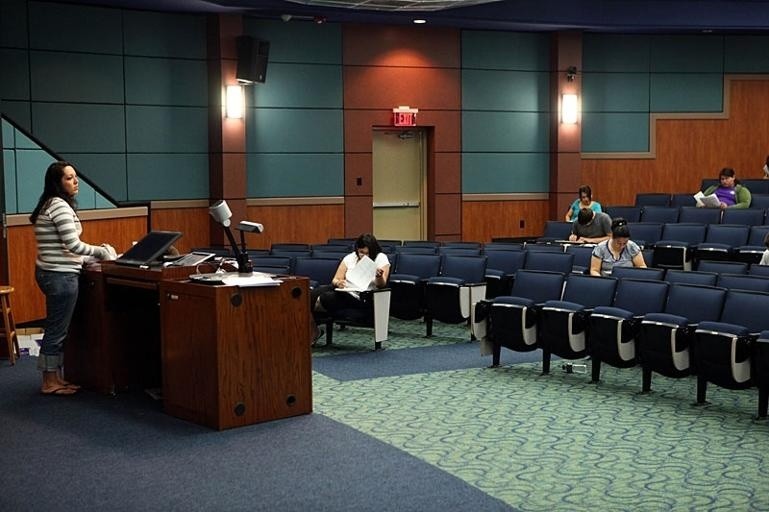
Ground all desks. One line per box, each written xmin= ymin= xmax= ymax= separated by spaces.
xmin=65 ymin=254 xmax=313 ymax=432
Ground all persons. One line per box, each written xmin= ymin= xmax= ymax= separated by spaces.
xmin=565 ymin=186 xmax=602 ymax=225
xmin=696 ymin=167 xmax=751 ymax=209
xmin=29 ymin=162 xmax=116 ymax=395
xmin=568 ymin=209 xmax=613 ymax=246
xmin=307 ymin=235 xmax=392 ymax=346
xmin=590 ymin=217 xmax=646 ymax=276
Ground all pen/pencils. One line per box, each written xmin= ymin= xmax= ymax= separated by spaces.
xmin=344 ymin=274 xmax=346 ymax=280
xmin=572 ymin=231 xmax=573 ymax=235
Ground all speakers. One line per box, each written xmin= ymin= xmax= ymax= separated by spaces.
xmin=236 ymin=38 xmax=271 ymax=84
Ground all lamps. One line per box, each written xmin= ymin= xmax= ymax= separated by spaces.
xmin=222 ymin=80 xmax=249 ymax=121
xmin=557 ymin=93 xmax=585 ymax=128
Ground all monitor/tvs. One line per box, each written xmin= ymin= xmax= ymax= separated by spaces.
xmin=175 ymin=251 xmax=215 ymax=267
xmin=115 ymin=231 xmax=182 ymax=265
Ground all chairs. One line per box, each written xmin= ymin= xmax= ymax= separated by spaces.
xmin=652 ymin=220 xmax=710 ymax=272
xmin=640 ymin=247 xmax=653 ymax=267
xmin=424 ymin=253 xmax=491 ymax=343
xmin=673 ymin=192 xmax=698 ymax=210
xmin=664 ymin=268 xmax=721 ymax=289
xmin=375 ymin=239 xmax=402 ymax=249
xmin=439 ymin=246 xmax=485 ymax=258
xmin=271 ymin=242 xmax=310 ymax=251
xmin=385 ymin=251 xmax=397 ymax=276
xmin=482 ymin=247 xmax=528 ymax=299
xmin=320 ymin=286 xmax=392 ymax=349
xmin=690 ymin=222 xmax=750 ymax=272
xmin=525 ymin=249 xmax=576 ymax=274
xmin=748 ymin=193 xmax=769 ymax=211
xmin=312 ymin=250 xmax=351 ymax=259
xmin=325 ymin=237 xmax=360 ymax=246
xmin=751 ymin=263 xmax=769 ymax=277
xmin=601 ymin=204 xmax=642 ymax=224
xmin=624 ymin=221 xmax=664 ymax=250
xmin=249 ymin=254 xmax=292 ymax=266
xmin=631 ymin=281 xmax=728 ymax=394
xmin=191 ymin=246 xmax=231 ymax=258
xmin=719 ymin=272 xmax=768 ymax=298
xmin=525 ymin=242 xmax=565 ymax=252
xmin=611 ymin=265 xmax=664 ymax=281
xmin=402 ymin=240 xmax=442 ymax=248
xmin=272 ymin=249 xmax=313 ymax=276
xmin=678 ymin=205 xmax=723 ymax=225
xmin=567 ymin=244 xmax=595 ymax=274
xmin=641 ymin=204 xmax=681 ymax=223
xmin=720 ymin=207 xmax=765 ymax=227
xmin=698 ymin=258 xmax=751 ymax=274
xmin=534 ymin=272 xmax=622 ymax=375
xmin=538 ymin=220 xmax=576 ymax=243
xmin=583 ymin=275 xmax=672 ymax=384
xmin=483 ymin=241 xmax=525 ymax=251
xmin=758 ymin=340 xmax=768 ymax=423
xmin=687 ymin=287 xmax=769 ymax=407
xmin=252 ymin=264 xmax=290 ymax=276
xmin=636 ymin=192 xmax=672 ymax=208
xmin=741 ymin=176 xmax=769 ymax=196
xmin=444 ymin=241 xmax=482 ymax=250
xmin=702 ymin=176 xmax=723 ymax=190
xmin=311 ymin=243 xmax=349 ymax=252
xmin=735 ymin=223 xmax=769 ymax=263
xmin=486 ymin=268 xmax=568 ymax=366
xmin=388 ymin=252 xmax=445 ymax=348
xmin=395 ymin=247 xmax=442 ymax=255
xmin=350 ymin=244 xmax=393 ymax=254
xmin=294 ymin=257 xmax=341 ymax=326
xmin=230 ymin=248 xmax=272 ymax=258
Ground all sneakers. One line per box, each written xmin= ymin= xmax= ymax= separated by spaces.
xmin=309 ymin=326 xmax=325 ymax=348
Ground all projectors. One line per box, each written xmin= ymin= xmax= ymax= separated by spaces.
xmin=189 ymin=200 xmax=281 ymax=285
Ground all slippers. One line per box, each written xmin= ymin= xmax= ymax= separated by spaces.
xmin=38 ymin=386 xmax=77 ymax=400
xmin=62 ymin=379 xmax=82 ymax=393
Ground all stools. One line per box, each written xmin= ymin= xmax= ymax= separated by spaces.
xmin=0 ymin=284 xmax=22 ymax=364
